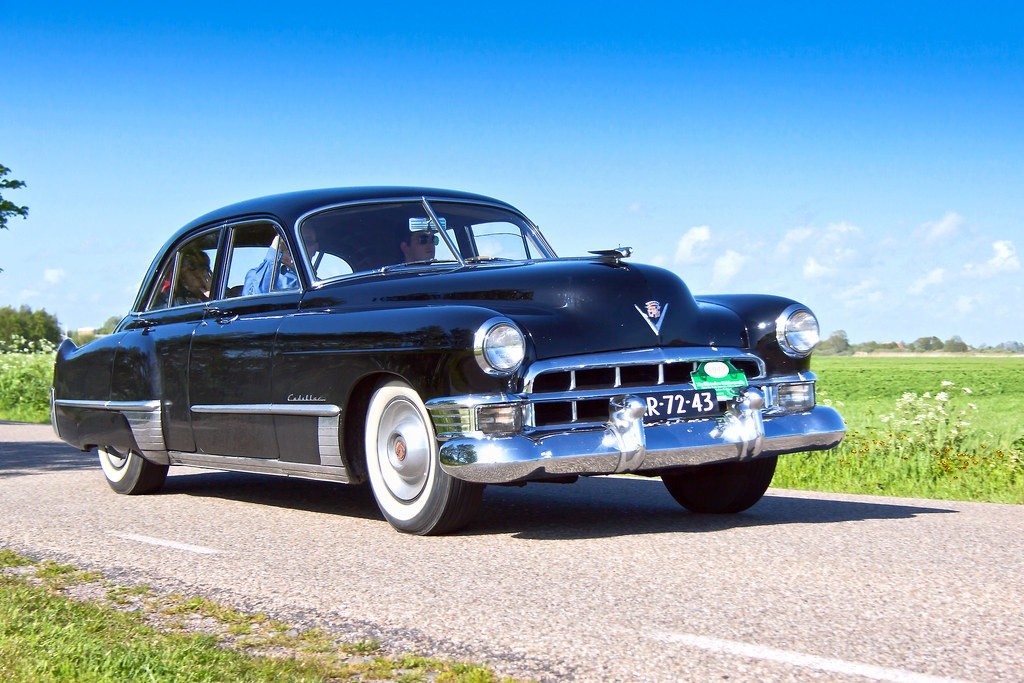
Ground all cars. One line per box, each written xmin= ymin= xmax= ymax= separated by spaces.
xmin=49 ymin=184 xmax=849 ymax=537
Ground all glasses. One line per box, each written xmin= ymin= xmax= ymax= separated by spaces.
xmin=410 ymin=234 xmax=439 ymax=245
xmin=305 ymin=239 xmax=318 ymax=246
xmin=181 ymin=263 xmax=210 ymax=271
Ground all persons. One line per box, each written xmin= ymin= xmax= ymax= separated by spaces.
xmin=242 ymin=216 xmax=320 ymax=297
xmin=150 ymin=247 xmax=216 ymax=314
xmin=393 ymin=213 xmax=438 ymax=265
